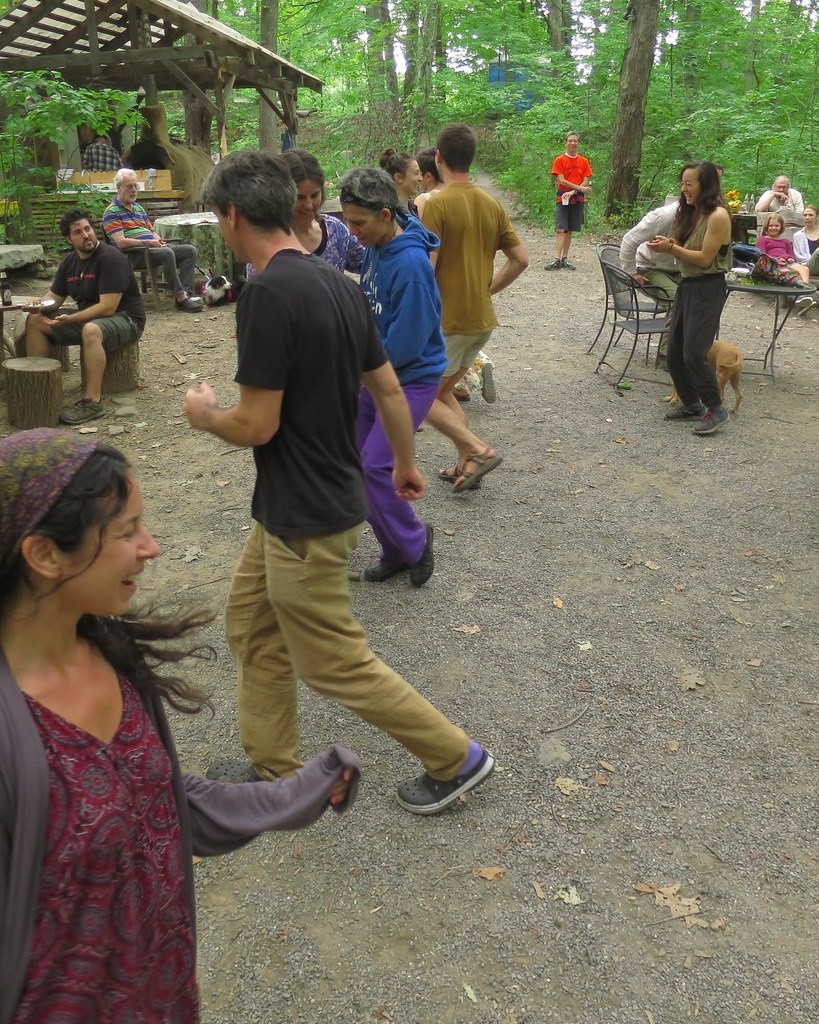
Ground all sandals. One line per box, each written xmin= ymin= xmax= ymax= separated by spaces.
xmin=436 ymin=465 xmax=482 ymax=489
xmin=453 ymin=444 xmax=502 ymax=493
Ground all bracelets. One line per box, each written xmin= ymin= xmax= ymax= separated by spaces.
xmin=669 ymin=243 xmax=674 ymax=254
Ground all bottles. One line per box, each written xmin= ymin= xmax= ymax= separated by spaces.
xmin=743 ymin=194 xmax=749 ymax=213
xmin=0 ymin=269 xmax=12 ymax=305
xmin=749 ymin=194 xmax=755 ymax=213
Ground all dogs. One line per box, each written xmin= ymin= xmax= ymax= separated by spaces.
xmin=662 ymin=317 xmax=743 ymax=413
xmin=201 ymin=276 xmax=233 ymax=306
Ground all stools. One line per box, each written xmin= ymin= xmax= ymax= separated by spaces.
xmin=15 ymin=312 xmax=70 ymax=374
xmin=2 ymin=357 xmax=63 ymax=431
xmin=80 ymin=340 xmax=140 ymax=395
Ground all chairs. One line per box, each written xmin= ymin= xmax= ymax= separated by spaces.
xmin=100 ymin=222 xmax=196 ymax=312
xmin=587 ymin=244 xmax=674 ymax=386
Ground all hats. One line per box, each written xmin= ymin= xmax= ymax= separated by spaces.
xmin=94 ymin=131 xmax=111 ymax=143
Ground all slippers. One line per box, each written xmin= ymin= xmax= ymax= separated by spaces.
xmin=206 ymin=759 xmax=256 ymax=784
xmin=395 ymin=743 xmax=495 ymax=815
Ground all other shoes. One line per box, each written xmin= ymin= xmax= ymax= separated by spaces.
xmin=544 ymin=258 xmax=576 ymax=271
xmin=795 ymin=293 xmax=817 ymax=316
xmin=364 ymin=559 xmax=404 ymax=582
xmin=174 ymin=296 xmax=203 ymax=313
xmin=481 ymin=362 xmax=497 ymax=404
xmin=409 ymin=522 xmax=434 ymax=586
xmin=659 ymin=352 xmax=669 ymax=371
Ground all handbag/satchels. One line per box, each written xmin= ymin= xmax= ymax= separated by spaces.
xmin=752 ymin=253 xmax=804 ymax=288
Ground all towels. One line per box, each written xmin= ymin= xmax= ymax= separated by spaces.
xmin=56 ymin=168 xmax=75 ymax=183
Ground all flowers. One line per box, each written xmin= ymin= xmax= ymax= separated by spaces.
xmin=725 ymin=190 xmax=741 ymax=202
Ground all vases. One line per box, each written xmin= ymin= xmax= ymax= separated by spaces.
xmin=727 ymin=200 xmax=742 ymax=214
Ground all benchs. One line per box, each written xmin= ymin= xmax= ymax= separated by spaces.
xmin=56 ymin=169 xmax=172 ymax=192
xmin=756 ymin=211 xmax=819 ymax=309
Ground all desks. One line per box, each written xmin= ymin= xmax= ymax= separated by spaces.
xmin=154 ymin=212 xmax=247 ymax=284
xmin=716 ymin=280 xmax=816 ymax=386
xmin=0 ymin=296 xmax=41 ymax=390
xmin=730 ymin=212 xmax=757 ymax=246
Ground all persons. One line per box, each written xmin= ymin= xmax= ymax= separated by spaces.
xmin=103 ymin=168 xmax=204 ymax=313
xmin=82 ymin=130 xmax=170 ymax=171
xmin=186 ymin=150 xmax=493 ymax=816
xmin=0 ymin=430 xmax=364 ymax=1024
xmin=619 ymin=162 xmax=733 ymax=435
xmin=244 ymin=123 xmax=528 ymax=587
xmin=755 ymin=176 xmax=819 ymax=310
xmin=25 ymin=211 xmax=147 ymax=424
xmin=544 ymin=131 xmax=593 ymax=270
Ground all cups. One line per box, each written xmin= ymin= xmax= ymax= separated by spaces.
xmin=562 ymin=197 xmax=570 ymax=205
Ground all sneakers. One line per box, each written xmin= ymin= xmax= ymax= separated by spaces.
xmin=692 ymin=407 xmax=731 ymax=434
xmin=59 ymin=399 xmax=106 ymax=425
xmin=665 ymin=404 xmax=706 ymax=420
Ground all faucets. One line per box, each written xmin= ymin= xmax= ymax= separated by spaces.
xmin=81 ymin=169 xmax=91 ymax=188
